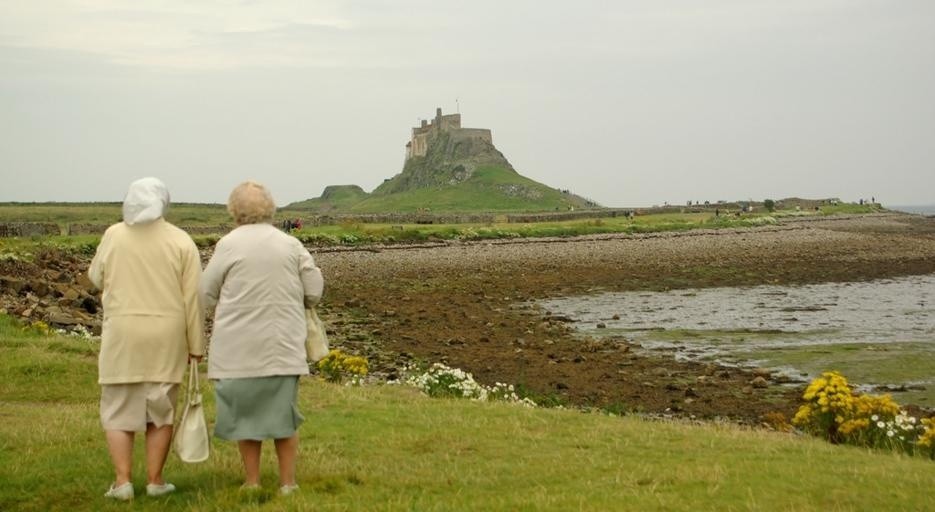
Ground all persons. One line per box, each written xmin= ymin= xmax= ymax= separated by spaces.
xmin=197 ymin=181 xmax=326 ymax=500
xmin=85 ymin=176 xmax=210 ymax=500
xmin=622 ymin=195 xmax=879 ymax=222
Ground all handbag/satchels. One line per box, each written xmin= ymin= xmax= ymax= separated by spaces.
xmin=305 ymin=306 xmax=329 ymax=362
xmin=173 ymin=393 xmax=210 ymax=464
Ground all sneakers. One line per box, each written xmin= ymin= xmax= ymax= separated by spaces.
xmin=278 ymin=484 xmax=299 ymax=495
xmin=105 ymin=482 xmax=134 ymax=500
xmin=147 ymin=483 xmax=176 ymax=496
xmin=240 ymin=482 xmax=263 ymax=491
